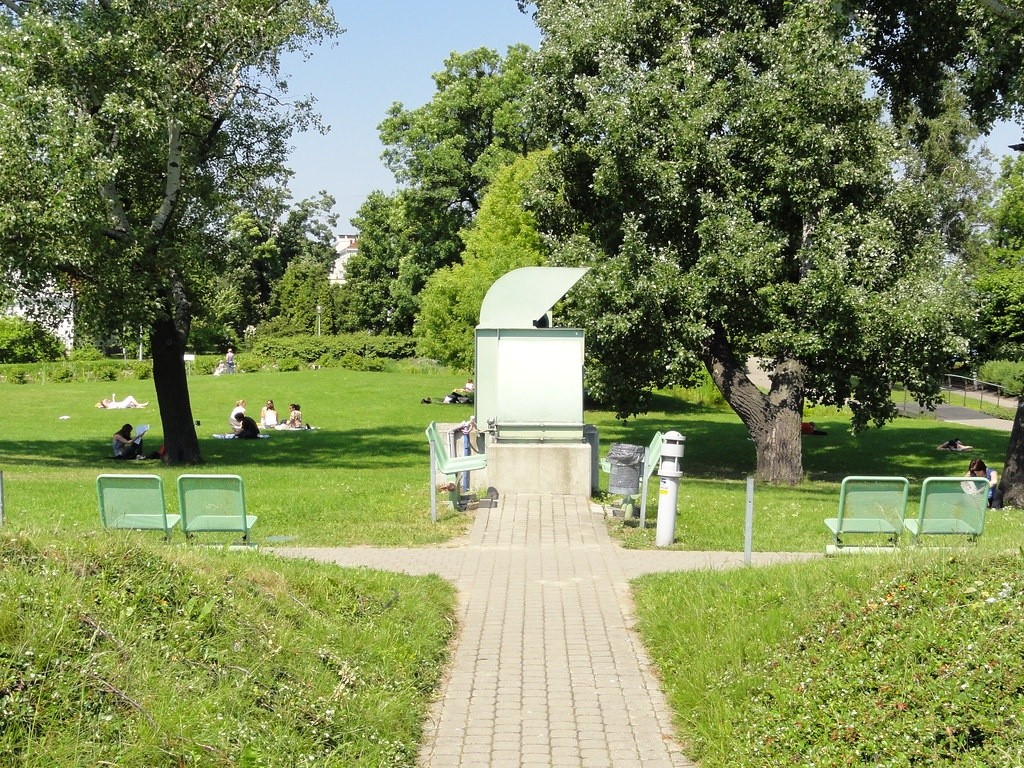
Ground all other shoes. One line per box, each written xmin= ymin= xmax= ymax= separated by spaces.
xmin=991 ymin=508 xmax=996 ymax=512
xmin=136 ymin=455 xmax=146 ymax=460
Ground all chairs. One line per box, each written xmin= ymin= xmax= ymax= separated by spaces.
xmin=903 ymin=477 xmax=990 ymax=550
xmin=177 ymin=474 xmax=258 ymax=545
xmin=96 ymin=474 xmax=180 ymax=544
xmin=824 ymin=476 xmax=909 ymax=548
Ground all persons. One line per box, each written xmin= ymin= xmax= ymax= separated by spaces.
xmin=466 ymin=379 xmax=474 ymax=392
xmin=234 ymin=412 xmax=260 ymax=437
xmin=219 ymin=360 xmax=224 ymax=368
xmin=937 ymin=438 xmax=973 ymax=451
xmin=230 ymin=400 xmax=246 ymax=429
xmin=226 ymin=349 xmax=234 ymax=374
xmin=280 ymin=404 xmax=302 ymax=427
xmin=99 ymin=393 xmax=148 ymax=409
xmin=113 ymin=424 xmax=146 ymax=460
xmin=962 ymin=459 xmax=1003 ymax=511
xmin=261 ymin=400 xmax=278 ymax=429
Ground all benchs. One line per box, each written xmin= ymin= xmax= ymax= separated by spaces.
xmin=425 ymin=420 xmax=488 ymax=525
xmin=601 ymin=431 xmax=667 ymax=521
